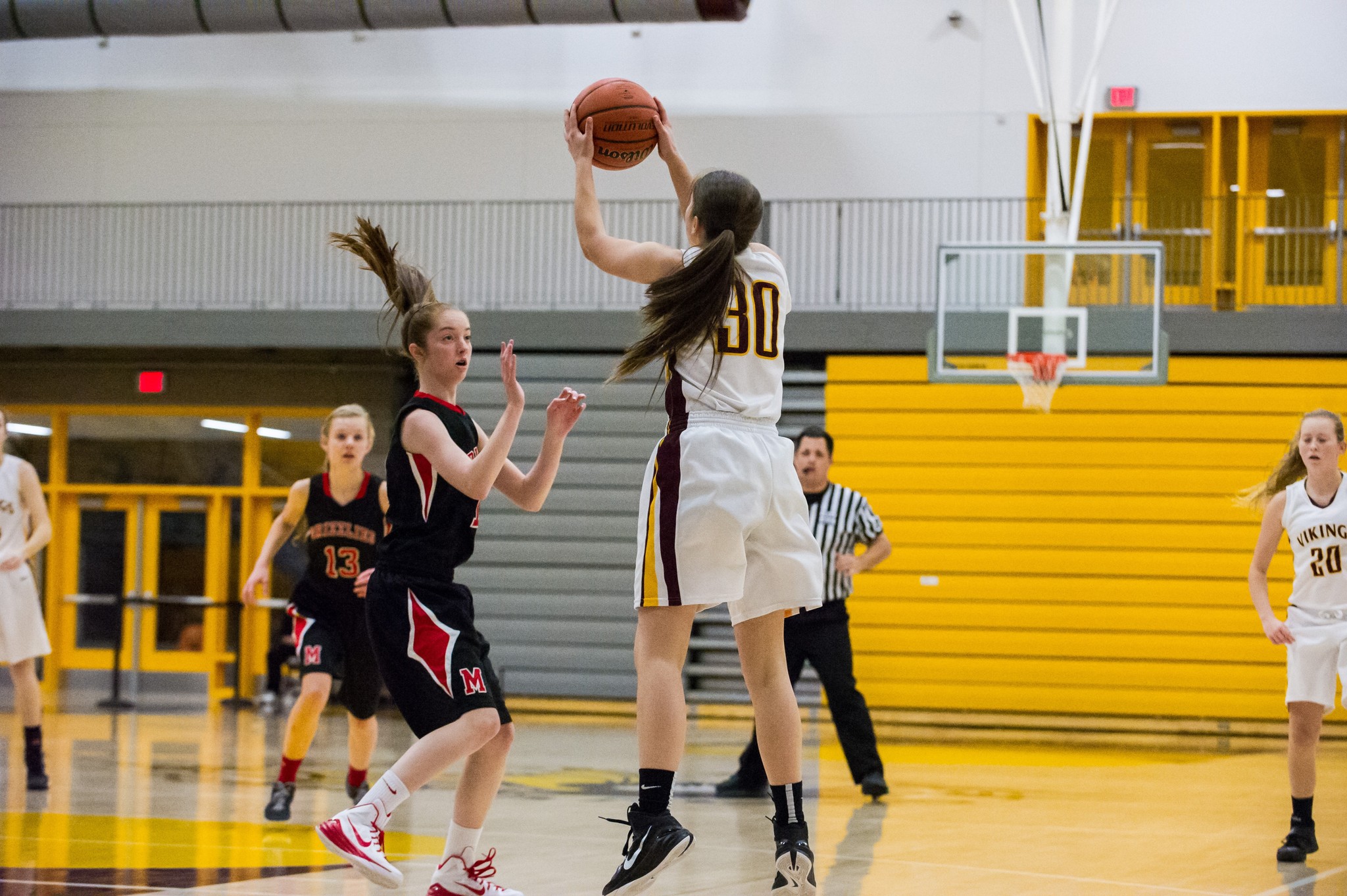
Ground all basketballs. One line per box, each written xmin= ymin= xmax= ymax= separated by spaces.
xmin=569 ymin=78 xmax=661 ymax=170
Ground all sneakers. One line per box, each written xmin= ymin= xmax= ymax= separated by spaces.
xmin=316 ymin=799 xmax=403 ymax=890
xmin=597 ymin=802 xmax=695 ymax=896
xmin=766 ymin=817 xmax=817 ymax=896
xmin=427 ymin=846 xmax=523 ymax=896
xmin=1276 ymin=816 xmax=1318 ymax=861
xmin=265 ymin=782 xmax=296 ymax=820
xmin=345 ymin=774 xmax=370 ymax=805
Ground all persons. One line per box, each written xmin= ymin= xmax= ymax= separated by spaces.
xmin=316 ymin=215 xmax=589 ymax=896
xmin=0 ymin=412 xmax=53 ymax=790
xmin=1248 ymin=409 xmax=1347 ymax=862
xmin=715 ymin=424 xmax=892 ymax=801
xmin=243 ymin=404 xmax=396 ymax=821
xmin=564 ymin=96 xmax=822 ymax=896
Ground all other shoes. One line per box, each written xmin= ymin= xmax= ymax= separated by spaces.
xmin=715 ymin=770 xmax=767 ymax=796
xmin=28 ymin=759 xmax=48 ymax=789
xmin=861 ymin=770 xmax=888 ymax=794
